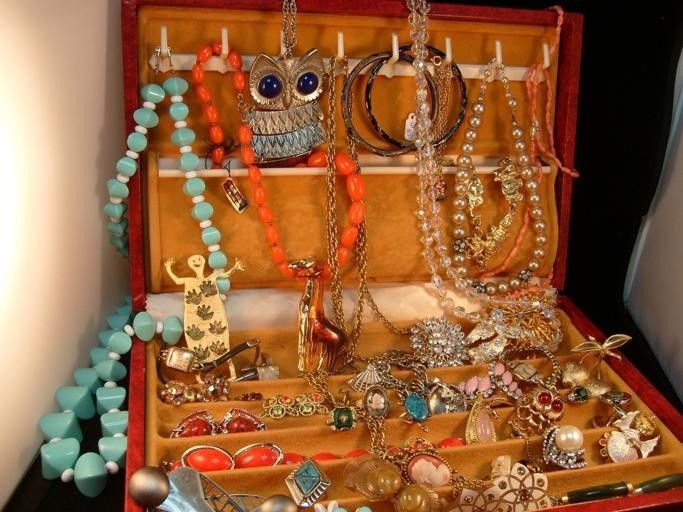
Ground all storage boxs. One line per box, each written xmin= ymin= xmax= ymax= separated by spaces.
xmin=122 ymin=0 xmax=681 ymax=512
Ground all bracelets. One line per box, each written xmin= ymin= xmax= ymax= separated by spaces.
xmin=340 ymin=52 xmax=438 ymax=158
xmin=364 ymin=45 xmax=467 ymax=151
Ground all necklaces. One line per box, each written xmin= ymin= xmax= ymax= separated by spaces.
xmin=408 ymin=0 xmax=562 ymax=352
xmin=476 ymin=6 xmax=580 ymax=299
xmin=298 ymin=53 xmax=486 ymax=497
xmin=249 ymin=1 xmax=327 ymax=164
xmin=190 ymin=42 xmax=368 ymax=278
xmin=39 ymin=44 xmax=230 ymax=497
xmin=452 ymin=59 xmax=546 ymax=295
